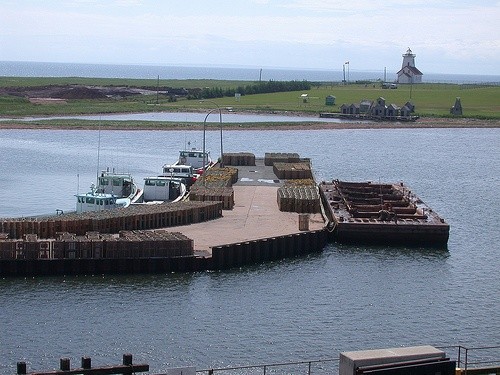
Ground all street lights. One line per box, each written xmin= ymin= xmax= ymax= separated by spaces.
xmin=203 ymin=106 xmax=233 ymax=185
xmin=200 ymin=99 xmax=225 ymax=166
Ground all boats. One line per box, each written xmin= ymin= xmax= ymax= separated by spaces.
xmin=76 ymin=172 xmax=137 ymax=208
xmin=131 ymin=177 xmax=187 ymax=206
xmin=163 ymin=148 xmax=211 ymax=185
